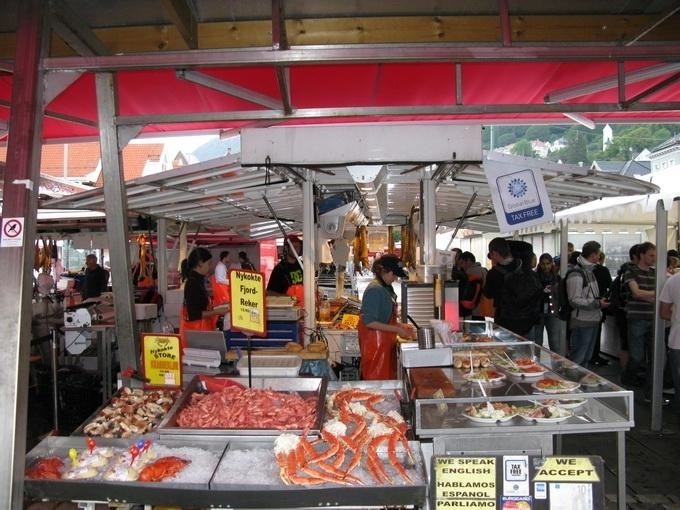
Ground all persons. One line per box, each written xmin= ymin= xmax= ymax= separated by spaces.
xmin=179 ymin=248 xmax=256 ymax=339
xmin=355 ymin=254 xmax=417 ymax=379
xmin=74 ymin=255 xmax=107 ymax=300
xmin=444 ymin=241 xmax=679 ymax=409
xmin=266 ymin=235 xmax=303 ymax=318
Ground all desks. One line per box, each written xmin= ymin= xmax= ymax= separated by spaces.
xmin=400 ymin=318 xmax=635 ymax=510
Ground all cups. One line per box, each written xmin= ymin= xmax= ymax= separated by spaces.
xmin=418 ymin=327 xmax=435 ymax=349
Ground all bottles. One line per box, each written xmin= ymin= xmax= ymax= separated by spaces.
xmin=318 ymin=294 xmax=330 ymax=323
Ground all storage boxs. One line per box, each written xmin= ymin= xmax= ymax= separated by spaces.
xmin=321 ymin=327 xmax=361 ymax=357
xmin=237 ymin=355 xmax=303 ymax=377
xmin=402 ymin=345 xmax=453 ymax=367
xmin=134 ymin=303 xmax=158 ymax=321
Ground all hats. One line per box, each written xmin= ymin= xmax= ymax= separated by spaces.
xmin=378 ymin=253 xmax=408 ymax=278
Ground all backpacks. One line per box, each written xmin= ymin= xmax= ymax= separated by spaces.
xmin=600 ymin=275 xmax=625 ymax=316
xmin=555 ymin=267 xmax=588 ymax=320
